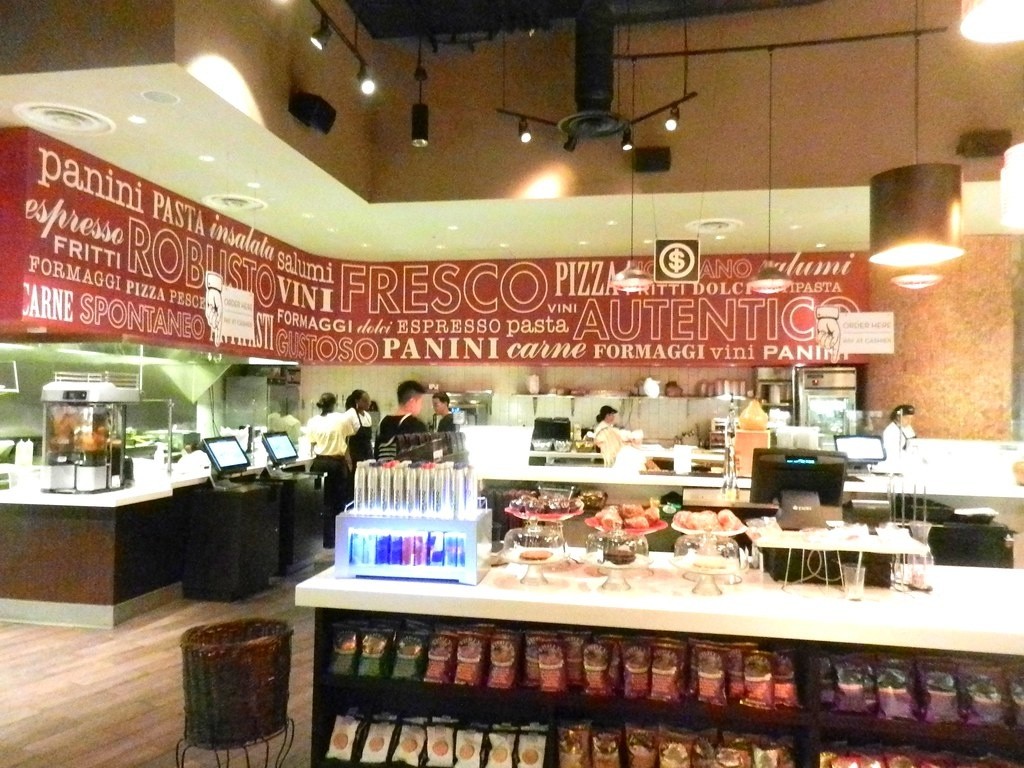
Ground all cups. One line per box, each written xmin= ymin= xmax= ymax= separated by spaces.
xmin=842 ymin=563 xmax=865 ymax=601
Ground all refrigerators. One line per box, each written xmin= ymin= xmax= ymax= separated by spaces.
xmin=795 ymin=367 xmax=857 ymax=450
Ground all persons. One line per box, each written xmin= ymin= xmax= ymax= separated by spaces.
xmin=307 ymin=393 xmax=355 ymax=549
xmin=431 ymin=392 xmax=457 ymax=432
xmin=883 ymin=405 xmax=917 ymax=474
xmin=374 ymin=381 xmax=433 ymax=462
xmin=346 ymin=389 xmax=374 ymax=473
xmin=593 ymin=406 xmax=643 ymax=468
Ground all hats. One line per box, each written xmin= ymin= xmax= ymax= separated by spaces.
xmin=600 ymin=406 xmax=617 ymax=415
xmin=894 ymin=405 xmax=916 ymax=415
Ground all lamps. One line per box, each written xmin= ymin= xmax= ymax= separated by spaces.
xmin=747 ymin=46 xmax=792 ymax=296
xmin=865 ymin=32 xmax=966 ymax=291
xmin=309 ymin=0 xmax=376 ymax=95
xmin=410 ymin=26 xmax=429 ymax=147
xmin=496 ymin=5 xmax=697 ymax=151
xmin=607 ymin=55 xmax=651 ymax=294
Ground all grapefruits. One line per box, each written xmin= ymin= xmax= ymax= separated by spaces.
xmin=739 ymin=398 xmax=768 ymax=430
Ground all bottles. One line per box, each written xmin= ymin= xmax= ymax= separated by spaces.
xmin=348 ymin=460 xmax=478 ymax=566
xmin=908 ymin=522 xmax=934 ymax=591
xmin=529 ymin=374 xmax=540 ymax=394
xmin=14 ymin=438 xmax=33 ymax=467
xmin=695 ymin=379 xmax=753 ymax=396
xmin=740 ymin=396 xmax=768 ymax=431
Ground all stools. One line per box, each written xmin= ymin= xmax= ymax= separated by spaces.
xmin=176 ymin=714 xmax=296 ymax=767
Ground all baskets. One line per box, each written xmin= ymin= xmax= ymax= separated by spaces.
xmin=180 ymin=618 xmax=294 ymax=748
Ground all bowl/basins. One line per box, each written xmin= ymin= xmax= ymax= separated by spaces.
xmin=575 ymin=440 xmax=594 ymax=452
xmin=531 ymin=439 xmax=553 ymax=451
xmin=554 ymin=439 xmax=573 ymax=452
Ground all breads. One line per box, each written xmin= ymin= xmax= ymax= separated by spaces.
xmin=511 ymin=492 xmax=582 ymax=515
xmin=671 ymin=508 xmax=744 ymax=534
xmin=594 ymin=502 xmax=659 ymax=530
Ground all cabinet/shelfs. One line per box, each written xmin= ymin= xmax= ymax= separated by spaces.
xmin=295 ymin=557 xmax=1024 ymax=768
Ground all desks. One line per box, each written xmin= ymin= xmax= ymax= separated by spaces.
xmin=470 ymin=436 xmax=1024 ymax=581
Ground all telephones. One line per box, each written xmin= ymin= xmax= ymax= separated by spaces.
xmin=895 ymin=494 xmax=954 ymax=524
